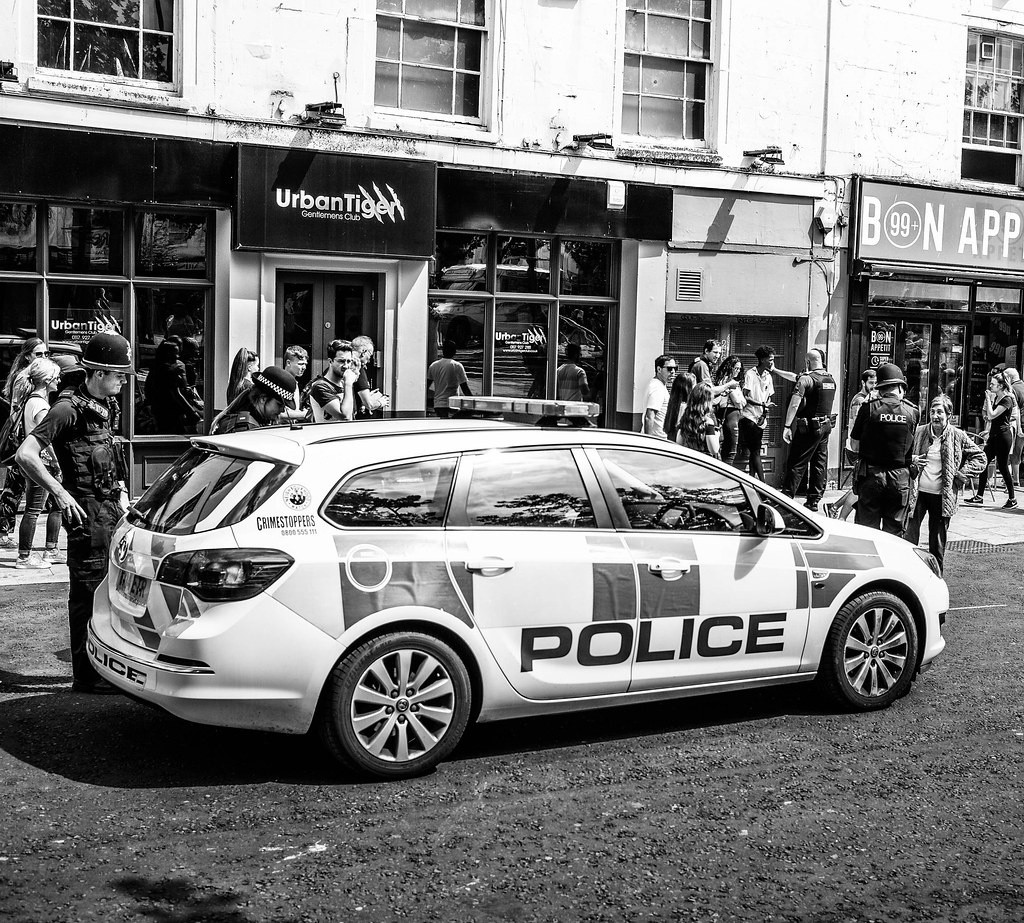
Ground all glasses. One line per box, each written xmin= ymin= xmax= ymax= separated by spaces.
xmin=364 ymin=349 xmax=374 ymax=356
xmin=32 ymin=349 xmax=51 ymax=358
xmin=44 ymin=376 xmax=61 ymax=381
xmin=662 ymin=365 xmax=680 ymax=371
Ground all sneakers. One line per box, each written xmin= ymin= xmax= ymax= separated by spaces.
xmin=1002 ymin=498 xmax=1019 ymax=509
xmin=15 ymin=549 xmax=52 ymax=569
xmin=43 ymin=546 xmax=67 ymax=564
xmin=824 ymin=503 xmax=838 ymax=519
xmin=963 ymin=495 xmax=984 ymax=504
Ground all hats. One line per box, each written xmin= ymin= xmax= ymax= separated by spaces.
xmin=251 ymin=366 xmax=297 ymax=410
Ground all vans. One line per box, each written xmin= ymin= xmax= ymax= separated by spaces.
xmin=433 ymin=266 xmax=551 ymax=350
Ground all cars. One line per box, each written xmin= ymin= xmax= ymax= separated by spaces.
xmin=86 ymin=396 xmax=950 ymax=777
xmin=0 ymin=328 xmax=159 ymax=435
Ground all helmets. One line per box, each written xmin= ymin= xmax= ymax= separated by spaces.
xmin=80 ymin=330 xmax=136 ymax=375
xmin=874 ymin=362 xmax=909 ymax=390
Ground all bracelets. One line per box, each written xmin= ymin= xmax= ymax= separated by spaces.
xmin=784 ymin=425 xmax=791 ymax=429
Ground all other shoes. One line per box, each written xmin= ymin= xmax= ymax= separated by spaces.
xmin=0 ymin=538 xmax=19 ymax=548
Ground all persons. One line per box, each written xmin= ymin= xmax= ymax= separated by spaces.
xmin=551 ymin=343 xmax=592 ymax=426
xmin=771 ymin=348 xmax=836 ymax=510
xmin=905 ymin=358 xmax=961 ymax=412
xmin=903 ymin=394 xmax=988 ymax=577
xmin=982 ymin=368 xmax=1024 ymax=488
xmin=681 ymin=381 xmax=722 ymax=463
xmin=640 ymin=353 xmax=679 ymax=443
xmin=424 ymin=339 xmax=475 ymax=416
xmin=227 ymin=348 xmax=260 ymax=407
xmin=209 ymin=366 xmax=296 ymax=437
xmin=280 ymin=336 xmax=389 ymax=424
xmin=687 ymin=340 xmax=778 ymax=483
xmin=964 ymin=373 xmax=1018 ymax=510
xmin=664 ymin=373 xmax=697 ymax=445
xmin=824 ymin=364 xmax=921 ymax=538
xmin=142 ymin=317 xmax=204 ymax=434
xmin=15 ymin=330 xmax=138 ymax=695
